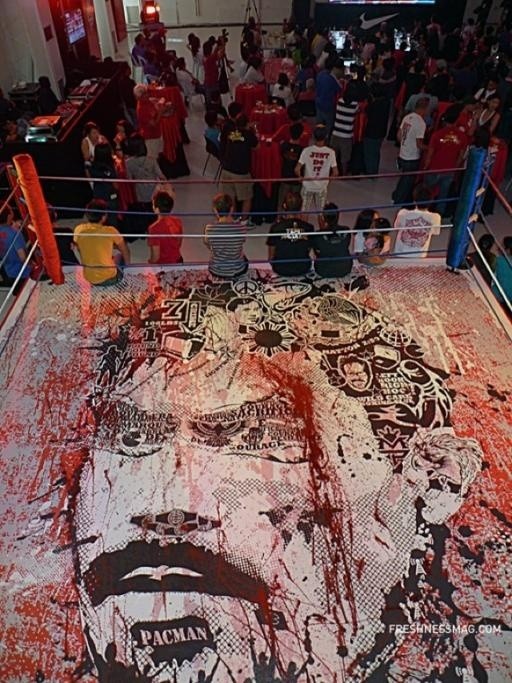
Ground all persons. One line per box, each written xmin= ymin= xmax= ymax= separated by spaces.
xmin=46 ymin=202 xmax=79 ymax=264
xmin=490 ymin=235 xmax=511 ymax=304
xmin=365 ymin=217 xmax=394 ymax=255
xmin=0 ymin=12 xmax=512 ymax=243
xmin=146 ymin=190 xmax=185 ymax=263
xmin=466 ymin=232 xmax=496 ymax=290
xmin=73 ymin=197 xmax=131 ymax=286
xmin=0 ymin=199 xmax=33 ymax=295
xmin=50 ymin=272 xmax=488 ymax=683
xmin=309 ymin=201 xmax=354 ymax=277
xmin=266 ymin=190 xmax=315 ymax=276
xmin=204 ymin=192 xmax=249 ymax=278
xmin=347 ymin=208 xmax=379 ymax=255
xmin=358 ymin=235 xmax=388 ymax=267
xmin=393 ymin=182 xmax=442 ymax=256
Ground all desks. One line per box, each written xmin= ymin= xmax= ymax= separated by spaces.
xmin=46 ymin=61 xmax=126 ymax=143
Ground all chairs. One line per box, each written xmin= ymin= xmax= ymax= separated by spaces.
xmin=127 ymin=49 xmax=319 ymax=191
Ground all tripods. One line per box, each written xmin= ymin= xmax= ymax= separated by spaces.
xmin=241 ymin=0 xmax=261 ymax=35
xmin=217 ymin=45 xmax=233 ymax=100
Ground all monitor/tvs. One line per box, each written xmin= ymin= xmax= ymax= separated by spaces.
xmin=63 ymin=6 xmax=87 ymax=45
xmin=393 ymin=33 xmax=411 ymax=51
xmin=329 ymin=31 xmax=354 ymax=50
